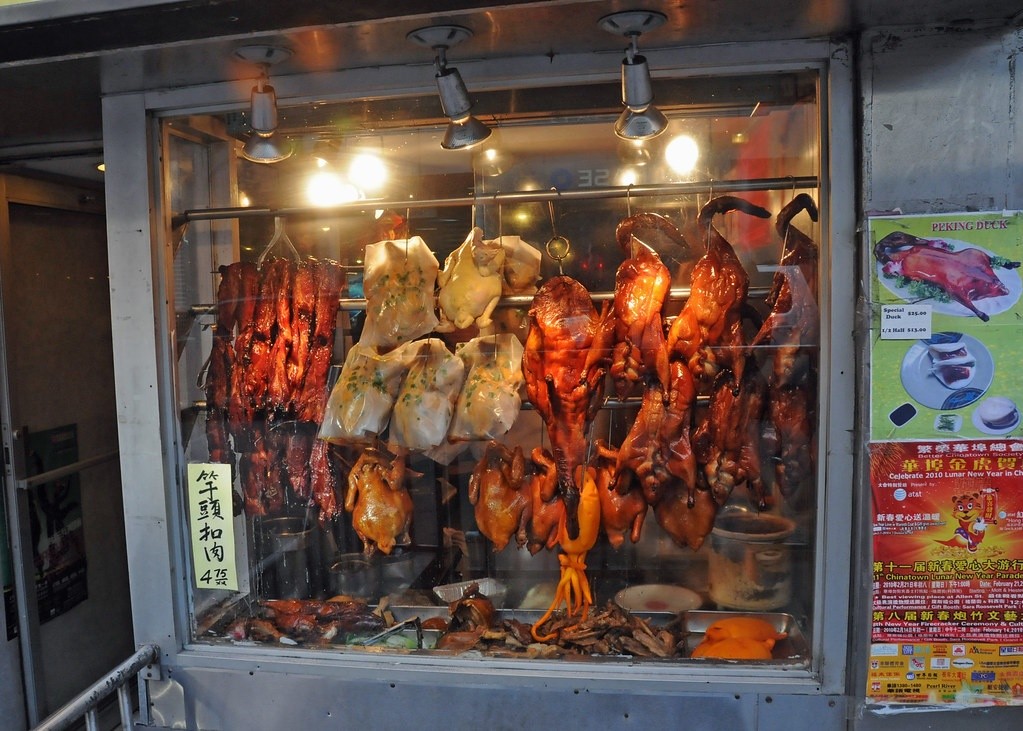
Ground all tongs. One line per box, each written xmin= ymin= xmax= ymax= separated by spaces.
xmin=362 ymin=616 xmax=424 ymax=649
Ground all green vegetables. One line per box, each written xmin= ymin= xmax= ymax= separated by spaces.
xmin=882 ymin=244 xmax=1009 ymax=303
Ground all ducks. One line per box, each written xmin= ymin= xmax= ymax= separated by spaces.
xmin=245 ymin=594 xmax=385 ymax=649
xmin=522 ymin=192 xmax=817 ymax=556
xmin=206 ymin=261 xmax=340 ymax=522
xmin=873 ymin=231 xmax=1021 ymax=322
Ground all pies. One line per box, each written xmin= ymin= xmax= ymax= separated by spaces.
xmin=928 ymin=343 xmax=976 ymax=390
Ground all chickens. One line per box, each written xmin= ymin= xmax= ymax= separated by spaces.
xmin=337 ymin=226 xmax=542 ymax=555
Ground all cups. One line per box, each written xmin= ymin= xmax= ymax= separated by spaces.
xmin=254 ymin=517 xmax=322 ymax=601
xmin=326 ymin=551 xmax=379 ymax=605
xmin=379 ymin=547 xmax=415 ymax=598
xmin=705 ymin=512 xmax=796 ymax=611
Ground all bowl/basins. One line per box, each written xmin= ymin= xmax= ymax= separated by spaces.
xmin=888 ymin=401 xmax=918 ymax=428
xmin=433 ymin=576 xmax=508 ymax=609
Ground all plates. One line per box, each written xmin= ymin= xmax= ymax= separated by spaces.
xmin=900 ymin=331 xmax=995 ymax=411
xmin=876 ymin=237 xmax=1023 ymax=317
xmin=971 ymin=405 xmax=1020 ymax=435
xmin=614 ymin=584 xmax=703 ymax=613
xmin=933 ymin=413 xmax=962 ymax=433
